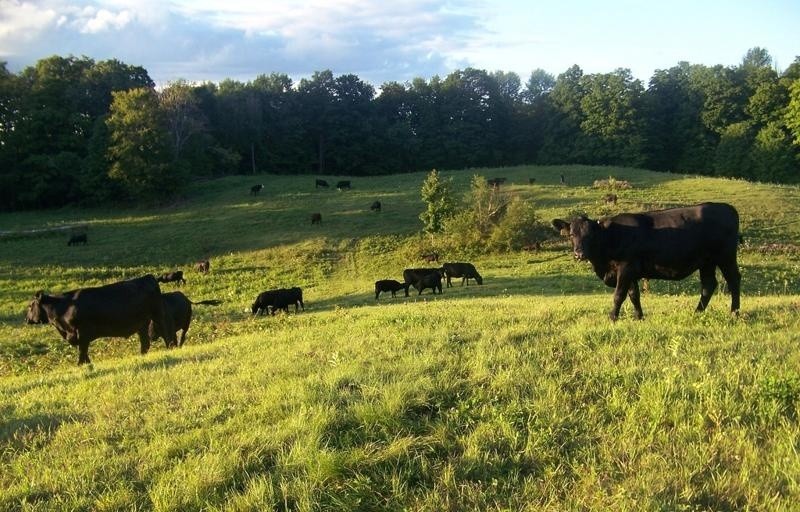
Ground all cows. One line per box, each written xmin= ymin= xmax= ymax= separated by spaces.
xmin=24 ymin=270 xmax=223 ymax=366
xmin=529 ymin=178 xmax=536 ymax=184
xmin=375 ymin=263 xmax=483 ymax=298
xmin=250 ymin=182 xmax=265 ymax=196
xmin=66 ymin=234 xmax=90 ymax=247
xmin=251 ymin=286 xmax=305 ymax=316
xmin=370 ymin=200 xmax=381 ymax=212
xmin=550 ymin=199 xmax=743 ymax=323
xmin=311 ymin=213 xmax=322 ymax=224
xmin=315 ymin=178 xmax=352 ymax=190
xmin=192 ymin=260 xmax=211 ymax=275
xmin=420 ymin=254 xmax=440 ymax=263
xmin=487 ymin=177 xmax=508 ymax=187
